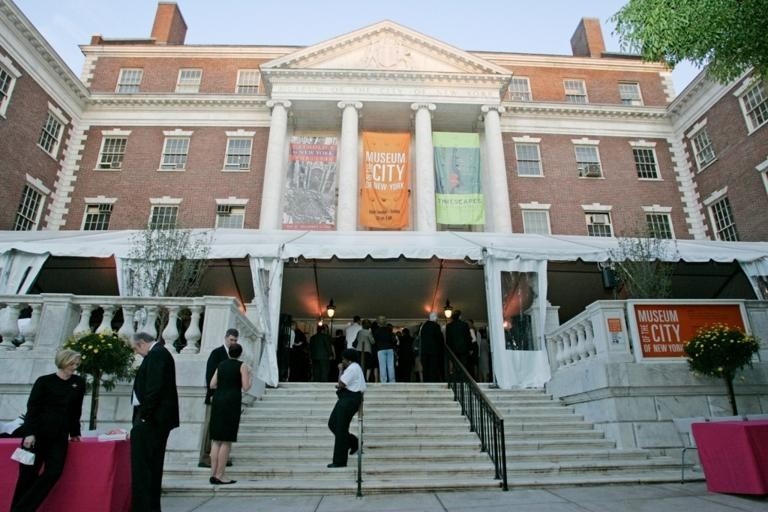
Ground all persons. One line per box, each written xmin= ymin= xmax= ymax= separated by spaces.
xmin=290 ymin=309 xmax=490 ymax=383
xmin=208 ymin=343 xmax=254 ymax=485
xmin=327 ymin=348 xmax=368 ymax=469
xmin=197 ymin=327 xmax=233 ymax=467
xmin=106 ymin=331 xmax=180 ymax=512
xmin=8 ymin=348 xmax=86 ymax=512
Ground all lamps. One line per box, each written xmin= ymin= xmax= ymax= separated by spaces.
xmin=326 ymin=298 xmax=335 ymax=319
xmin=318 ymin=315 xmax=325 ymax=327
xmin=443 ymin=297 xmax=454 ymax=319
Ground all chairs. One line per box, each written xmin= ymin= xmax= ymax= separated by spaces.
xmin=672 ymin=413 xmax=768 ymax=496
xmin=81 ymin=430 xmax=98 ymax=438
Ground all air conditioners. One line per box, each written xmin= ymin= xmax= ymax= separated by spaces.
xmin=110 ymin=162 xmax=121 ymax=169
xmin=585 ymin=165 xmax=600 ymax=177
xmin=591 ymin=214 xmax=606 ymax=224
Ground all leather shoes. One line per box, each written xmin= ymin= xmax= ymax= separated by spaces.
xmin=349 ymin=441 xmax=363 ymax=455
xmin=210 ymin=477 xmax=237 ymax=484
xmin=327 ymin=463 xmax=347 ymax=468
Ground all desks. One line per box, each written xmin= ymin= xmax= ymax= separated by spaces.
xmin=0 ymin=435 xmax=133 ymax=512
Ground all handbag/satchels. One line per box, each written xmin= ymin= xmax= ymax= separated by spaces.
xmin=352 ymin=339 xmax=358 ymax=348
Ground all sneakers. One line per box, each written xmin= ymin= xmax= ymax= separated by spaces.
xmin=198 ymin=462 xmax=211 ymax=467
xmin=226 ymin=461 xmax=233 ymax=466
